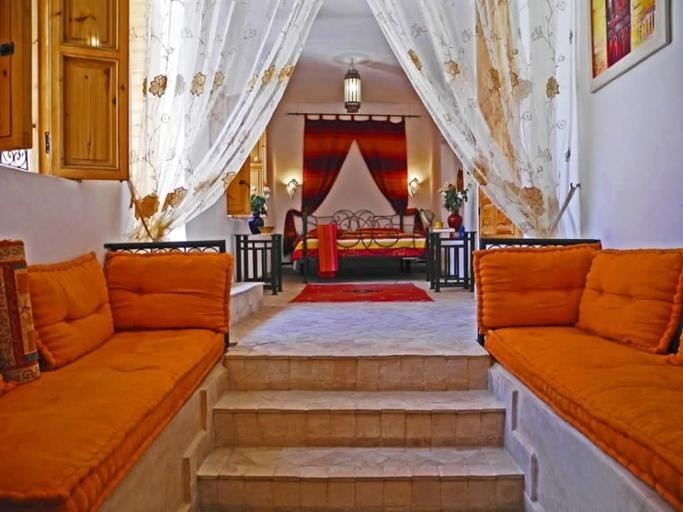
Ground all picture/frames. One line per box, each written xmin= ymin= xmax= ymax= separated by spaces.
xmin=585 ymin=0 xmax=674 ymax=94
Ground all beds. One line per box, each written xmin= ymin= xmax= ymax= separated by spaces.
xmin=300 ymin=208 xmax=430 ymax=283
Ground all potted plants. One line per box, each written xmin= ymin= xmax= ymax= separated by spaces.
xmin=247 ymin=194 xmax=267 ymax=233
xmin=437 ymin=182 xmax=472 ymax=230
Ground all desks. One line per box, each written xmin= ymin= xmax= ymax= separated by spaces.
xmin=234 ymin=232 xmax=283 ymax=295
xmin=430 ymin=230 xmax=476 ymax=293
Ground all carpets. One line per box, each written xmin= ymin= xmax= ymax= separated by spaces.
xmin=288 ymin=280 xmax=434 ymax=303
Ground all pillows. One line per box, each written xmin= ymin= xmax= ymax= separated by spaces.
xmin=477 ymin=243 xmax=683 ymax=353
xmin=20 ymin=249 xmax=234 ymax=364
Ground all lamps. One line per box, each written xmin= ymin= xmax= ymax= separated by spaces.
xmin=287 ymin=178 xmax=297 ymax=201
xmin=408 ymin=178 xmax=418 ymax=199
xmin=343 ymin=58 xmax=361 ymax=114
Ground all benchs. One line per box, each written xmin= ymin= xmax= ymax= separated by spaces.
xmin=472 ymin=237 xmax=683 ymax=502
xmin=1 ymin=240 xmax=228 ymax=512
